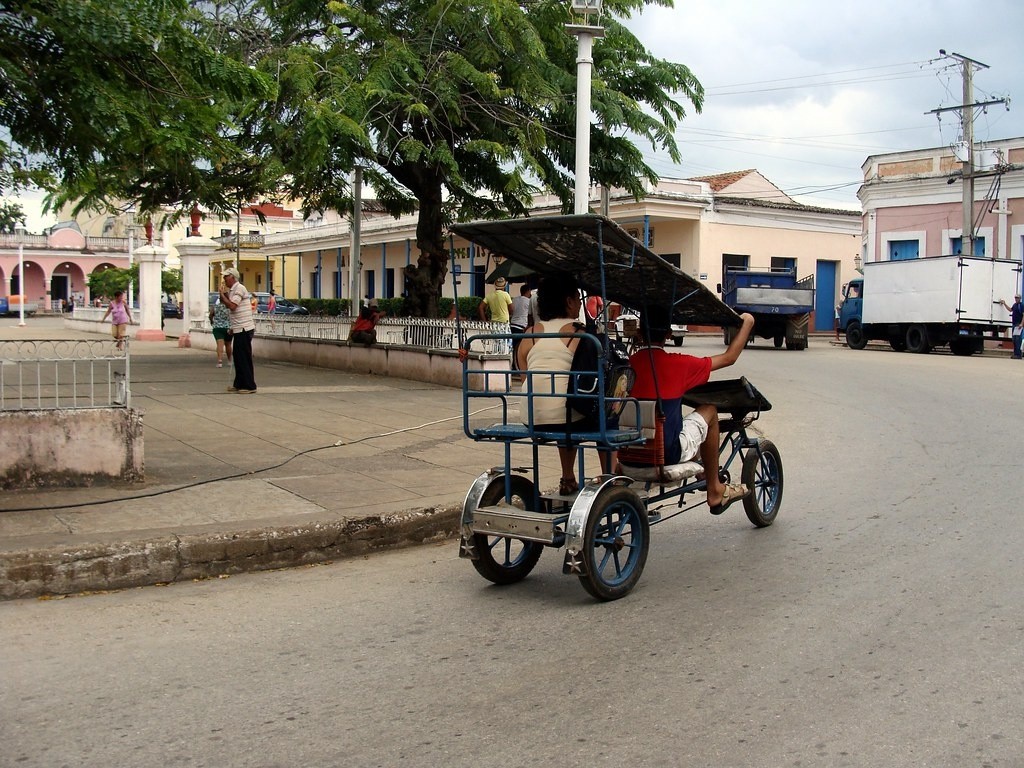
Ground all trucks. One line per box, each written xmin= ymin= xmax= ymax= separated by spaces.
xmin=717 ymin=265 xmax=815 ymax=350
xmin=0 ymin=298 xmax=38 ymax=318
xmin=838 ymin=254 xmax=1024 ymax=355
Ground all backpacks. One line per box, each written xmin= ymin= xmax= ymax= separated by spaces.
xmin=565 ymin=325 xmax=636 ymax=422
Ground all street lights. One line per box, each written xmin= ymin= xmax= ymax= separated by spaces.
xmin=14 ymin=220 xmax=27 ymax=326
xmin=125 ymin=204 xmax=136 ymax=312
xmin=564 ymin=0 xmax=607 ymax=214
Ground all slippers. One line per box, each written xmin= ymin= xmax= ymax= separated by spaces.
xmin=710 ymin=483 xmax=751 ymax=515
xmin=699 ymin=465 xmax=724 ymax=491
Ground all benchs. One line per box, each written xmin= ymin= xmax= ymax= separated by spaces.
xmin=462 ymin=332 xmax=647 ymax=486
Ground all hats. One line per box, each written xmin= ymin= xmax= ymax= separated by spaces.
xmin=220 ymin=267 xmax=240 ymax=278
xmin=370 ymin=298 xmax=378 ymax=307
xmin=494 ymin=276 xmax=508 ymax=288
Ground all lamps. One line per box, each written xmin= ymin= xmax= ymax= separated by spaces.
xmin=314 ymin=265 xmax=323 ymax=272
xmin=360 ymin=261 xmax=363 ymax=270
xmin=491 ymin=254 xmax=502 ymax=264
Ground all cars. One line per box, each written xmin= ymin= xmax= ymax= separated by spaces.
xmin=161 ymin=303 xmax=183 ymax=319
xmin=209 ymin=292 xmax=222 ymax=310
xmin=614 ymin=306 xmax=689 ymax=346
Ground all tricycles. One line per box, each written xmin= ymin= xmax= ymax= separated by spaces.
xmin=446 ymin=213 xmax=785 ymax=603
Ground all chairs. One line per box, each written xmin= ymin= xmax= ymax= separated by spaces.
xmin=618 ymin=399 xmax=705 ymax=485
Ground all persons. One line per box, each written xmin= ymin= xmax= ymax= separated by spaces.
xmin=79 ymin=296 xmax=83 ymax=307
xmin=100 ymin=291 xmax=136 ymax=351
xmin=268 ymin=290 xmax=275 ymax=332
xmin=479 ymin=277 xmax=541 ymax=376
xmin=1001 ymin=294 xmax=1024 ymax=359
xmin=209 ymin=292 xmax=233 ymax=368
xmin=93 ymin=295 xmax=114 ymax=308
xmin=218 ymin=268 xmax=257 ymax=394
xmin=62 ymin=296 xmax=74 ymax=312
xmin=363 ymin=295 xmax=378 ymax=308
xmin=619 ymin=307 xmax=754 ymax=515
xmin=351 ymin=299 xmax=387 ymax=347
xmin=251 ymin=292 xmax=257 ymax=331
xmin=835 ymin=300 xmax=842 ymax=341
xmin=587 ymin=295 xmax=603 ymax=318
xmin=517 ymin=276 xmax=619 ymax=496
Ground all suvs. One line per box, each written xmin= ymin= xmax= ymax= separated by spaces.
xmin=253 ymin=292 xmax=309 ymax=316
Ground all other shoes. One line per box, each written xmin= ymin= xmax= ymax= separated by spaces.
xmin=1011 ymin=356 xmax=1022 ymax=359
xmin=238 ymin=388 xmax=257 ymax=394
xmin=559 ymin=477 xmax=579 ymax=495
xmin=228 ymin=387 xmax=238 ymax=391
xmin=228 ymin=362 xmax=232 ymax=366
xmin=216 ymin=361 xmax=223 ymax=368
